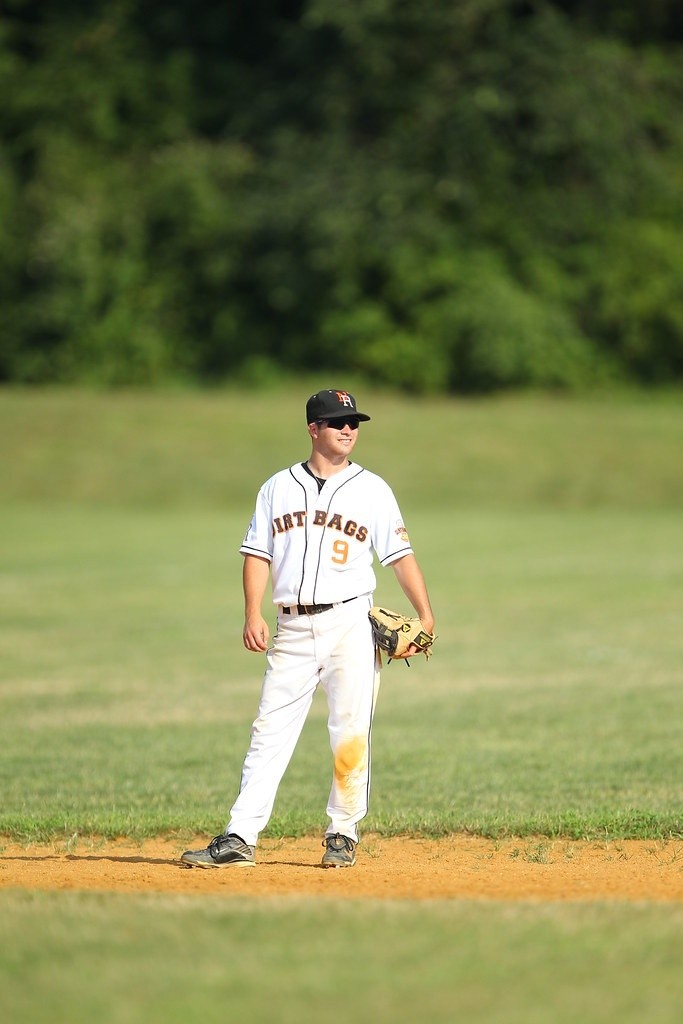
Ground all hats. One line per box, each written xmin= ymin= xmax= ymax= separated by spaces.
xmin=306 ymin=389 xmax=370 ymax=426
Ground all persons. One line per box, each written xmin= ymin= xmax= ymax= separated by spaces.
xmin=181 ymin=389 xmax=437 ymax=870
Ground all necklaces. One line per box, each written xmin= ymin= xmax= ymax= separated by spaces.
xmin=308 ymin=461 xmax=349 ymax=487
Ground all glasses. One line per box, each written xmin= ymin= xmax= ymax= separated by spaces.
xmin=315 ymin=417 xmax=359 ymax=430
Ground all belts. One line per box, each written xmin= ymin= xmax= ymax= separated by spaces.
xmin=283 ymin=597 xmax=358 ymax=615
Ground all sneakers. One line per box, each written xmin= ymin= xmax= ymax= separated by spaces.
xmin=322 ymin=832 xmax=356 ymax=869
xmin=181 ymin=832 xmax=256 ymax=869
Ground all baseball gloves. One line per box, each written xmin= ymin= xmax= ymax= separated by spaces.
xmin=368 ymin=606 xmax=438 ymax=661
